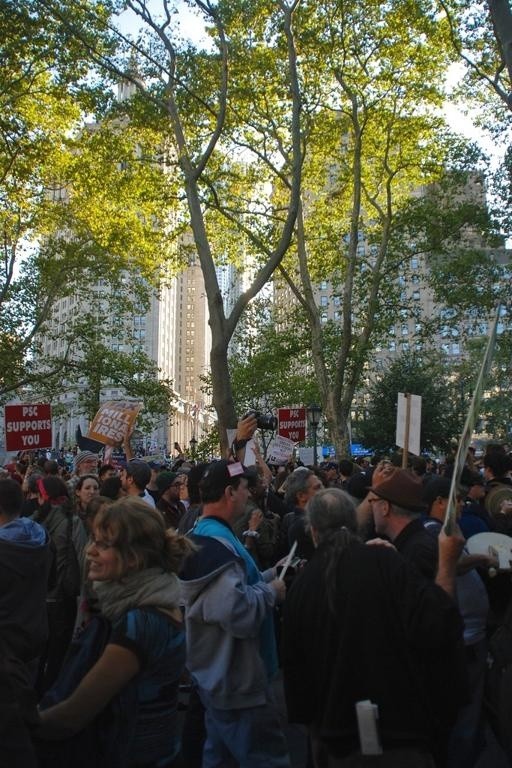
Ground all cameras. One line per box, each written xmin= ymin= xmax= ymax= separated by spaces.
xmin=241 ymin=408 xmax=278 ymax=431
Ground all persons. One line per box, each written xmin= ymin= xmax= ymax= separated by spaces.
xmin=1 ymin=411 xmax=511 ymax=767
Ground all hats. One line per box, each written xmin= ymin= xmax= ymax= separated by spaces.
xmin=35 ymin=449 xmax=191 ymax=505
xmin=365 ymin=458 xmax=429 ymax=513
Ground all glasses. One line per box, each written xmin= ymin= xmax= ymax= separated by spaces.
xmin=88 ymin=530 xmax=119 ymax=552
